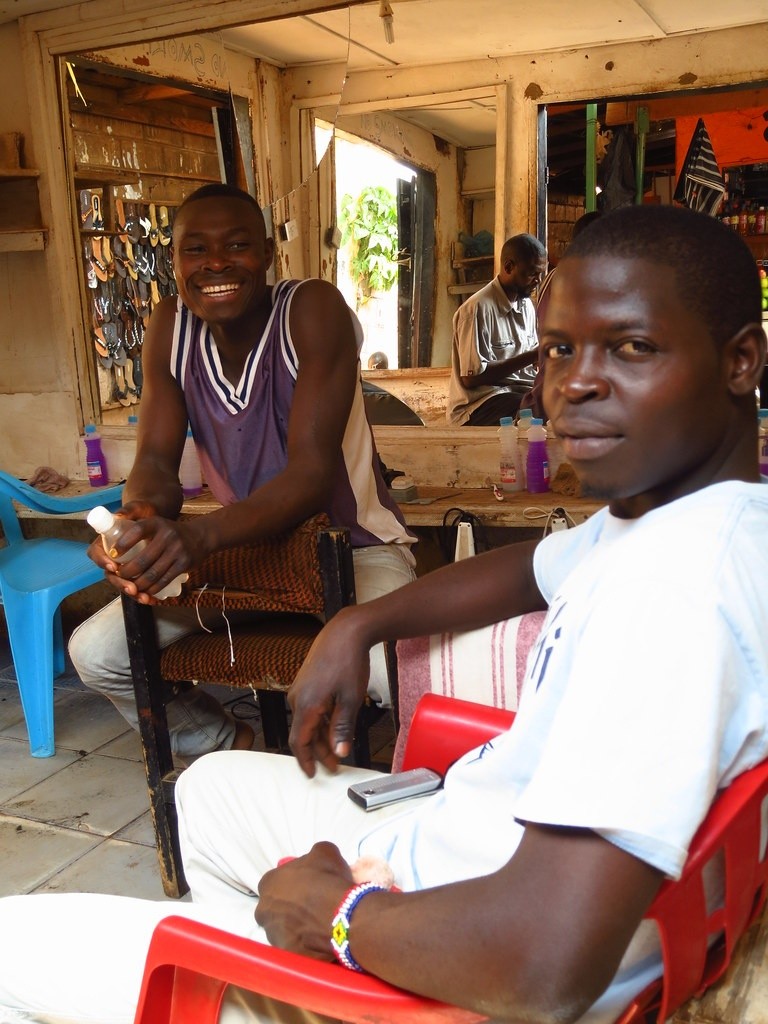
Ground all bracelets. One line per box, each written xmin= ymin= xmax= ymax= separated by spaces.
xmin=327 ymin=879 xmax=403 ymax=974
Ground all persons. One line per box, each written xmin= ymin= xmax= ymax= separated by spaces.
xmin=512 ymin=212 xmax=607 ymax=428
xmin=172 ymin=202 xmax=768 ymax=1024
xmin=65 ymin=188 xmax=421 ymax=775
xmin=445 ymin=234 xmax=548 ymax=428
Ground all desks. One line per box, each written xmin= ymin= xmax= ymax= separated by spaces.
xmin=10 ymin=478 xmax=124 ymax=521
xmin=176 ymin=484 xmax=611 ymax=531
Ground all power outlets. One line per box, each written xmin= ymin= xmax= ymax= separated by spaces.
xmin=327 ymin=226 xmax=343 ymax=249
xmin=281 ymin=218 xmax=299 ymax=242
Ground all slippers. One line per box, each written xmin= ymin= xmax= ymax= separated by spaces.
xmin=77 ymin=190 xmax=180 ymax=406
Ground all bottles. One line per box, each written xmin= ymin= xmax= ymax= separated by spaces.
xmin=498 ymin=416 xmax=525 ymax=494
xmin=526 ymin=419 xmax=550 ymax=493
xmin=82 ymin=424 xmax=109 ymax=487
xmin=518 ymin=408 xmax=536 ymax=429
xmin=84 ymin=506 xmax=190 ymax=601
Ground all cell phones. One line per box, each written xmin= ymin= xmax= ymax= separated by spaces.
xmin=347 ymin=767 xmax=445 ymax=811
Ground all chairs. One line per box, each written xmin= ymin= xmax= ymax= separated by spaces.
xmin=120 ymin=524 xmax=375 ymax=897
xmin=0 ymin=470 xmax=126 ymax=759
xmin=135 ymin=690 xmax=768 ymax=1024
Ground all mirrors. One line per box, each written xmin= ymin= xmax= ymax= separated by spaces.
xmin=12 ymin=0 xmax=767 ymax=440
